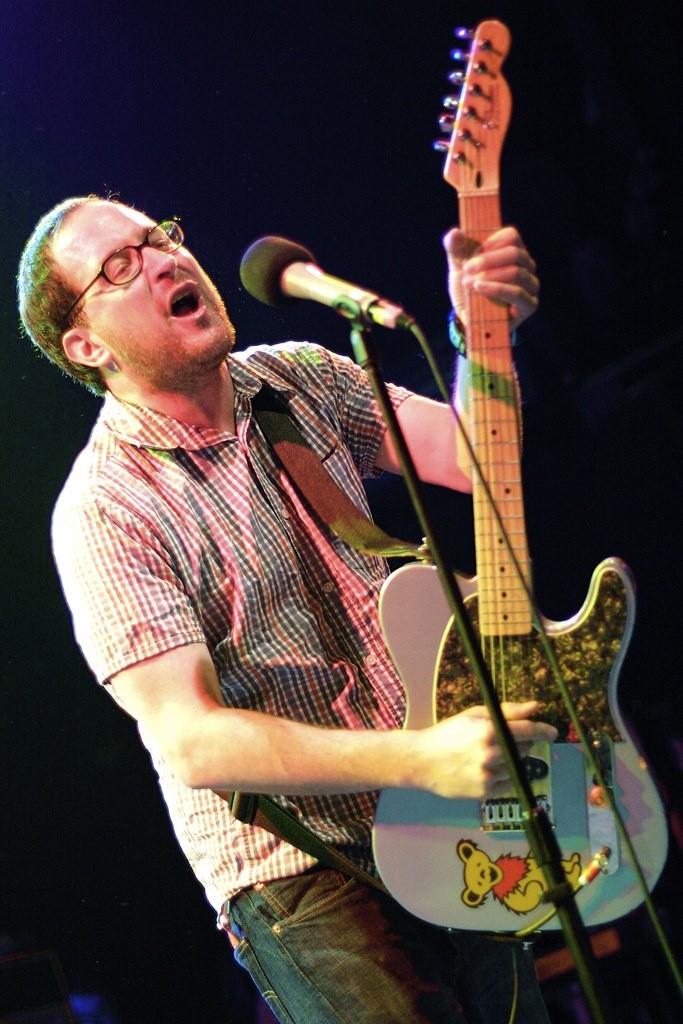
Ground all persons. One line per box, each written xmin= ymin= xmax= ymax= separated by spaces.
xmin=16 ymin=195 xmax=559 ymax=1024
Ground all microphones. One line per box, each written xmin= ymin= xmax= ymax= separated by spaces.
xmin=239 ymin=233 xmax=409 ymax=334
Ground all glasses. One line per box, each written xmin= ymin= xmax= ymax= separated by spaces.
xmin=60 ymin=220 xmax=185 ymax=332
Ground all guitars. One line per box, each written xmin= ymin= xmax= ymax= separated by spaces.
xmin=369 ymin=17 xmax=670 ymax=934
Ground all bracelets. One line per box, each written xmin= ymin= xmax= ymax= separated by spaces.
xmin=449 ymin=312 xmax=518 ymax=359
